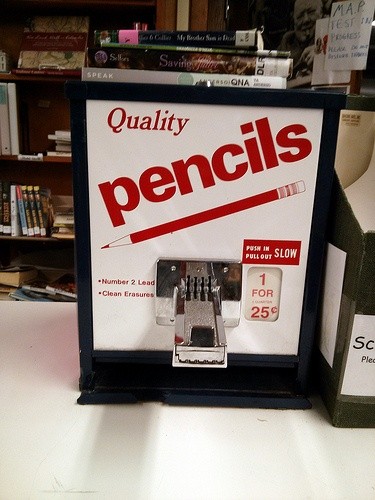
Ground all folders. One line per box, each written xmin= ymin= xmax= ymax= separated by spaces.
xmin=0 ymin=82 xmax=19 ymax=155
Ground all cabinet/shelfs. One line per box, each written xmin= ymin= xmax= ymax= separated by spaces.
xmin=0 ymin=0 xmax=227 ymax=293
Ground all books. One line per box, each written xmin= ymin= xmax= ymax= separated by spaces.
xmin=81 ymin=30 xmax=294 ymax=90
xmin=0 ymin=266 xmax=77 ymax=302
xmin=48 ymin=130 xmax=71 ymax=157
xmin=0 ymin=184 xmax=75 ymax=239
